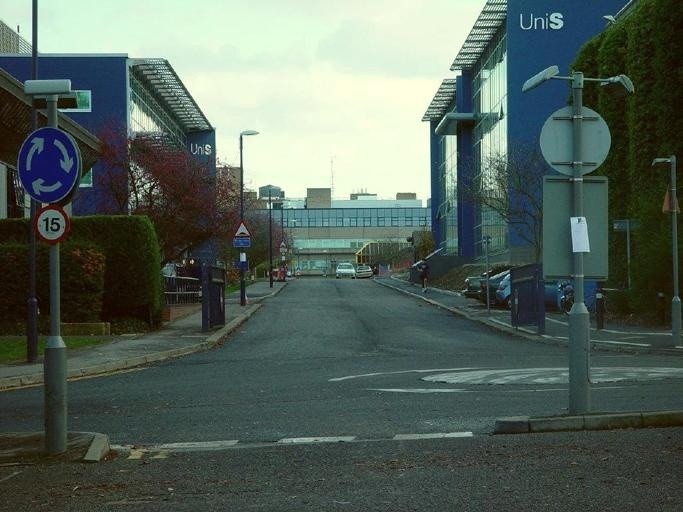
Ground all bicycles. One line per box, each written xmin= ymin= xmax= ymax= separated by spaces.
xmin=584 ymin=289 xmax=618 ymax=317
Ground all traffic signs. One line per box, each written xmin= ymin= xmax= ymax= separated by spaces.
xmin=35 ymin=208 xmax=71 ymax=243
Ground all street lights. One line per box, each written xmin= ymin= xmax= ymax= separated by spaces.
xmin=268 ymin=184 xmax=297 ymax=290
xmin=239 ymin=129 xmax=258 ymax=304
xmin=522 ymin=67 xmax=636 ymax=414
xmin=652 ymin=157 xmax=682 ymax=344
xmin=24 ymin=80 xmax=78 ymax=451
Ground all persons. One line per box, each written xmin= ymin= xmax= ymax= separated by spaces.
xmin=417 ymin=259 xmax=429 ymax=293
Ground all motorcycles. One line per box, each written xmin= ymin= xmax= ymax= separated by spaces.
xmin=556 ymin=279 xmax=575 ymax=316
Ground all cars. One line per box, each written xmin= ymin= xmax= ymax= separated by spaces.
xmin=464 ymin=264 xmax=561 ymax=309
xmin=336 ymin=262 xmax=374 ymax=279
